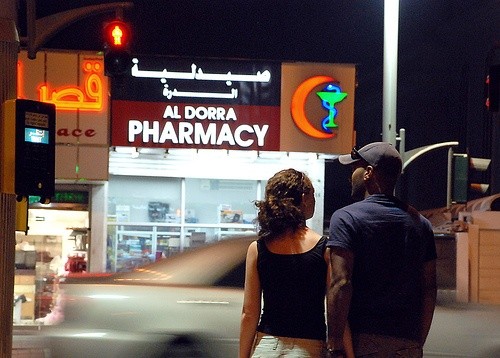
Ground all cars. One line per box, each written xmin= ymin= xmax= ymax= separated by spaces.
xmin=220 ymin=209 xmax=244 ymax=222
xmin=39 ymin=236 xmax=500 ymax=358
xmin=242 ymin=219 xmax=255 ymax=231
xmin=116 ymin=238 xmax=153 ymax=267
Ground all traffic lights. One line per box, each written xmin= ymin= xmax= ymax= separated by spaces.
xmin=480 ymin=72 xmax=494 ymax=113
xmin=100 ymin=10 xmax=136 ymax=80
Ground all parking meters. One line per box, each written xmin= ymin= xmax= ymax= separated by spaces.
xmin=3 ymin=98 xmax=57 ymax=201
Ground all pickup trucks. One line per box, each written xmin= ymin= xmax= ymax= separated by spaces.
xmin=185 ymin=210 xmax=199 ymax=223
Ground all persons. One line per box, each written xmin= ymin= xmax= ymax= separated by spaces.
xmin=323 ymin=143 xmax=437 ymax=358
xmin=239 ymin=169 xmax=355 ymax=358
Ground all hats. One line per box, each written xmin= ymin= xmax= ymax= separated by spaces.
xmin=338 ymin=141 xmax=402 ymax=179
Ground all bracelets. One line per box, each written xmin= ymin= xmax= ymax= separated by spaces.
xmin=326 ymin=347 xmax=344 ymax=355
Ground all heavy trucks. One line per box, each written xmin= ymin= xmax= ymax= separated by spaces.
xmin=148 ymin=202 xmax=169 ymax=221
